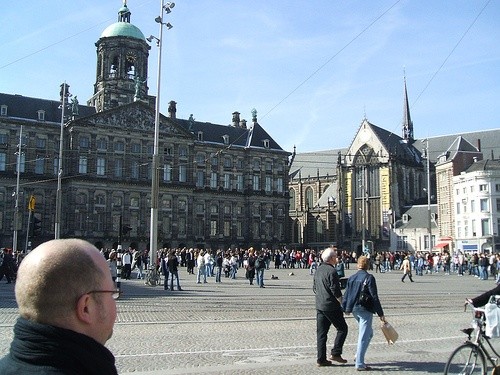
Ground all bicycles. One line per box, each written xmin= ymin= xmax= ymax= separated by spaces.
xmin=144 ymin=264 xmax=161 ymax=287
xmin=443 ymin=294 xmax=500 ymax=375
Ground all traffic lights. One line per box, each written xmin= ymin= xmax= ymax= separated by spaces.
xmin=28 ymin=212 xmax=43 ymax=237
xmin=123 ymin=226 xmax=133 ymax=234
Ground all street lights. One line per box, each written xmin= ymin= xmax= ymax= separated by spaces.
xmin=12 ymin=125 xmax=28 ymax=258
xmin=420 ymin=135 xmax=432 ymax=252
xmin=148 ymin=0 xmax=176 ymax=272
xmin=54 ymin=81 xmax=74 ymax=240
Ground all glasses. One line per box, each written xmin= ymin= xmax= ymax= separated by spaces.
xmin=86 ymin=288 xmax=120 ymax=299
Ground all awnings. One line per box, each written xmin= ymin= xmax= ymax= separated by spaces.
xmin=433 ymin=243 xmax=449 ymax=250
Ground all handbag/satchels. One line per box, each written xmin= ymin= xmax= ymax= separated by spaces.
xmin=358 ymin=274 xmax=377 ymax=314
xmin=381 ymin=320 xmax=398 ymax=344
xmin=484 ymin=295 xmax=500 ymax=338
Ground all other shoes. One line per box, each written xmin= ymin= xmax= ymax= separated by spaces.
xmin=329 ymin=355 xmax=347 ymax=363
xmin=357 ymin=367 xmax=371 ymax=370
xmin=317 ymin=359 xmax=332 ymax=365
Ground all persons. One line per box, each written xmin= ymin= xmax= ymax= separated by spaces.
xmin=0 ymin=247 xmax=28 ymax=284
xmin=414 ymin=251 xmax=452 ymax=276
xmin=451 ymin=248 xmax=500 ymax=281
xmin=222 ymin=247 xmax=273 ymax=288
xmin=274 ymin=249 xmax=324 ymax=275
xmin=100 ymin=247 xmax=148 ymax=282
xmin=368 ymin=250 xmax=415 ymax=273
xmin=400 ymin=256 xmax=415 ymax=283
xmin=189 ymin=114 xmax=196 ymax=132
xmin=0 ymin=238 xmax=123 ymax=375
xmin=312 ymin=247 xmax=348 ymax=366
xmin=341 ymin=256 xmax=385 ymax=371
xmin=158 ymin=248 xmax=224 ymax=291
xmin=71 ymin=95 xmax=79 ymax=115
xmin=465 ymin=284 xmax=500 ymax=308
xmin=334 ymin=251 xmax=358 ymax=278
